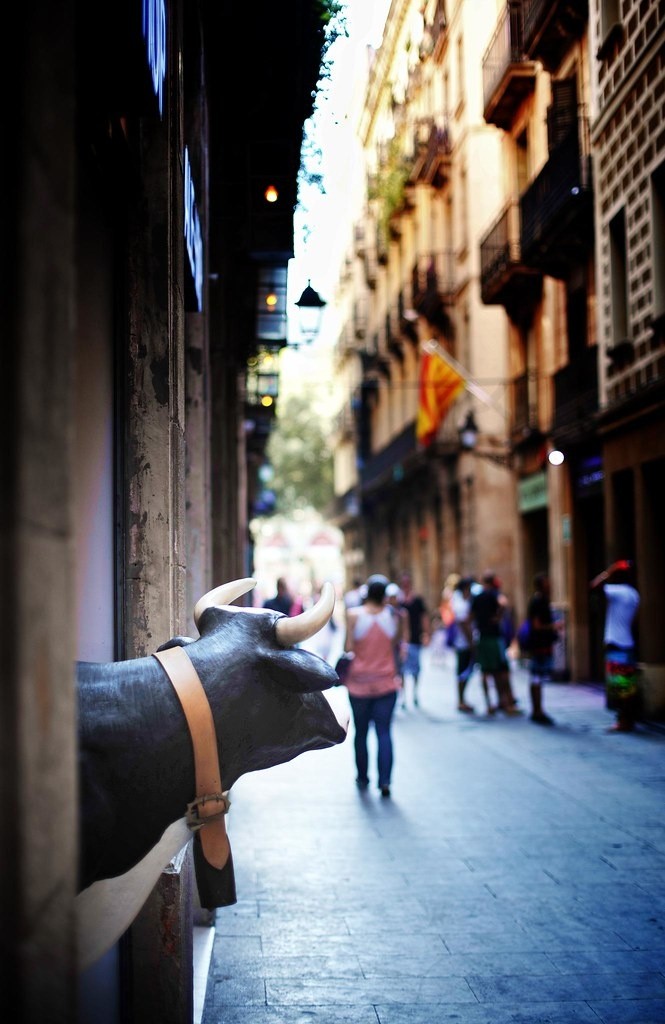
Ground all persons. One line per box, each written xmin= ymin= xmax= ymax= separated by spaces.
xmin=263 ymin=569 xmax=525 ymax=714
xmin=590 ymin=550 xmax=653 ymax=733
xmin=343 ymin=574 xmax=404 ymax=797
xmin=517 ymin=574 xmax=565 ymax=726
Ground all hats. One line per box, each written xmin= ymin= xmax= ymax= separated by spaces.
xmin=610 ymin=559 xmax=630 ymax=571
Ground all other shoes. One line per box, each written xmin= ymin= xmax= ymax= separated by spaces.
xmin=380 ymin=783 xmax=391 ymax=798
xmin=612 ymin=723 xmax=633 ymax=731
xmin=356 ymin=777 xmax=370 ymax=786
xmin=530 ymin=712 xmax=552 ymax=725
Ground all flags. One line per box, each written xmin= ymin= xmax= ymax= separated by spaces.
xmin=416 ymin=354 xmax=468 ymax=447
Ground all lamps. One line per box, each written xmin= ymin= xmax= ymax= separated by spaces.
xmin=286 ymin=278 xmax=326 ymax=351
xmin=456 ymin=409 xmax=511 ymax=473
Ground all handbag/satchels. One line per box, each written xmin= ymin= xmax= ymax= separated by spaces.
xmin=453 ymin=619 xmax=481 ymax=651
xmin=517 ymin=619 xmax=531 ymax=647
xmin=438 ymin=601 xmax=456 ymax=627
xmin=332 ymin=651 xmax=354 ymax=688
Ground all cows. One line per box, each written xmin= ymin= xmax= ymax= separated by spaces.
xmin=76 ymin=576 xmax=353 ymax=969
